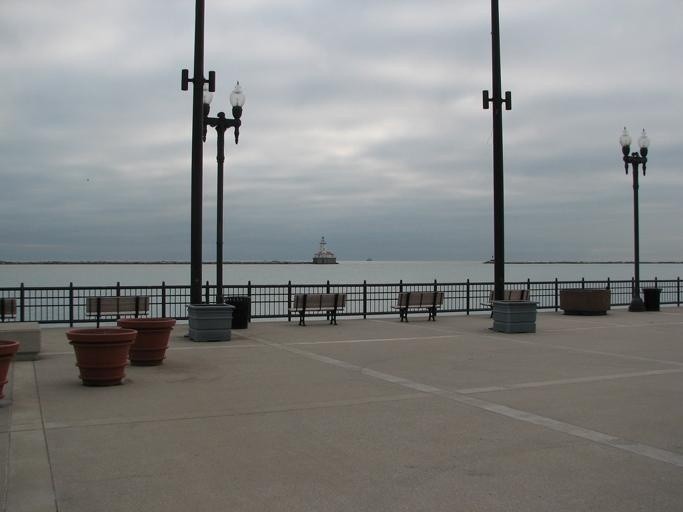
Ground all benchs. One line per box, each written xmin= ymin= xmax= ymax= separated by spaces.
xmin=480 ymin=290 xmax=531 ymax=317
xmin=288 ymin=293 xmax=346 ymax=326
xmin=0 ymin=298 xmax=17 ymax=323
xmin=86 ymin=296 xmax=150 ymax=328
xmin=391 ymin=291 xmax=445 ymax=323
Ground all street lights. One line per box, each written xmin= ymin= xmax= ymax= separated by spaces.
xmin=618 ymin=123 xmax=652 ymax=312
xmin=202 ymin=72 xmax=246 ymax=304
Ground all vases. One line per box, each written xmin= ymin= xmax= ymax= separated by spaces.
xmin=65 ymin=328 xmax=138 ymax=387
xmin=0 ymin=340 xmax=19 ymax=400
xmin=117 ymin=318 xmax=176 ymax=367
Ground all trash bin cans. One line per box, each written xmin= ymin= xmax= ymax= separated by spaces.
xmin=641 ymin=288 xmax=662 ymax=311
xmin=224 ymin=297 xmax=248 ymax=329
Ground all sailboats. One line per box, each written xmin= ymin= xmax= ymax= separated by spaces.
xmin=311 ymin=235 xmax=337 ymax=264
